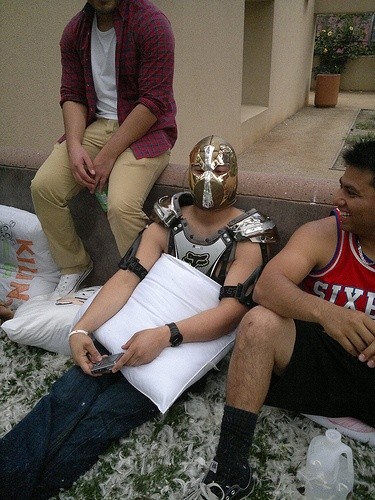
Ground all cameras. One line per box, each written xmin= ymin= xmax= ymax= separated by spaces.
xmin=90 ymin=353 xmax=123 ymax=373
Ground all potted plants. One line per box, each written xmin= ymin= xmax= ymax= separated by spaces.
xmin=312 ymin=63 xmax=345 ymax=108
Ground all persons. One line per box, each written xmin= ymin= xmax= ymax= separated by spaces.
xmin=30 ymin=0 xmax=177 ymax=303
xmin=0 ymin=135 xmax=281 ymax=500
xmin=182 ymin=139 xmax=375 ymax=500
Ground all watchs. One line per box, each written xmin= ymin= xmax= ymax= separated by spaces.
xmin=165 ymin=323 xmax=183 ymax=347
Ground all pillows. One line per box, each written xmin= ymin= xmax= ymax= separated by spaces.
xmin=0 ymin=204 xmax=236 ymax=414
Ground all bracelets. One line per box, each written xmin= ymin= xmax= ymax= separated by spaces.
xmin=66 ymin=330 xmax=89 ymax=340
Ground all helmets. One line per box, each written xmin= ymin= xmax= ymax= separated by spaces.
xmin=189 ymin=134 xmax=238 ymax=211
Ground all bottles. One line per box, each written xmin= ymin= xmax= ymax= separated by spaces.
xmin=304 ymin=429 xmax=354 ymax=500
xmin=89 ymin=172 xmax=108 ymax=212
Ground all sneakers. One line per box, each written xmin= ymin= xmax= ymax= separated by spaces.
xmin=180 ymin=456 xmax=255 ymax=500
xmin=52 ymin=260 xmax=94 ymax=295
